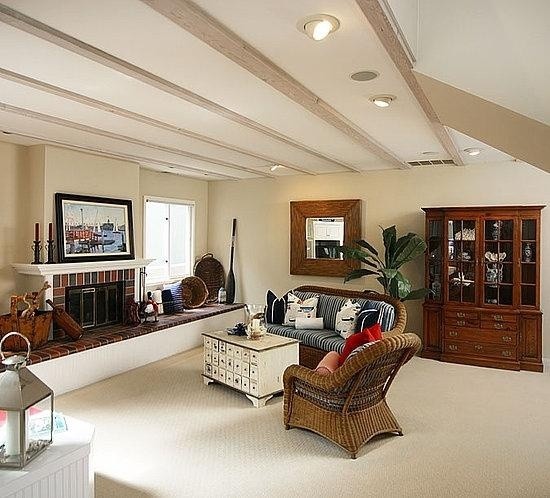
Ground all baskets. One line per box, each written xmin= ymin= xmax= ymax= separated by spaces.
xmin=193 ymin=252 xmax=225 ymax=309
xmin=182 ymin=276 xmax=209 ymax=310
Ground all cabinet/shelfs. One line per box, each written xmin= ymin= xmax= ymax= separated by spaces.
xmin=416 ymin=205 xmax=545 ymax=373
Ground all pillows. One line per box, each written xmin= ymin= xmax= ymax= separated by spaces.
xmin=356 ymin=300 xmax=385 ymax=332
xmin=282 ymin=293 xmax=320 ymax=327
xmin=339 ymin=323 xmax=383 ymax=367
xmin=336 ymin=299 xmax=362 ymax=340
xmin=265 ymin=289 xmax=294 ymax=325
xmin=296 ymin=317 xmax=324 ymax=329
xmin=315 ymin=351 xmax=340 ymax=374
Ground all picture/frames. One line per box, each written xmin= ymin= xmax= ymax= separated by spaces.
xmin=54 ymin=192 xmax=135 ymax=262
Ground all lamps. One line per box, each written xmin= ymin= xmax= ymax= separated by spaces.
xmin=369 ymin=95 xmax=397 ymax=107
xmin=297 ymin=14 xmax=340 ymax=41
xmin=0 ymin=331 xmax=53 ymax=469
xmin=465 ymin=147 xmax=482 ymax=156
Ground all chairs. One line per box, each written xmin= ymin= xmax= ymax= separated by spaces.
xmin=283 ymin=332 xmax=421 ymax=459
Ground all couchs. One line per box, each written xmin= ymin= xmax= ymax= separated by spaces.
xmin=256 ymin=284 xmax=407 ymax=371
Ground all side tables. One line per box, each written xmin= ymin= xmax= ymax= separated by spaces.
xmin=1 ymin=402 xmax=95 ymax=497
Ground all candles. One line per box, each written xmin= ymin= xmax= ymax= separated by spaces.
xmin=48 ymin=222 xmax=55 ymax=239
xmin=34 ymin=222 xmax=39 ymax=240
